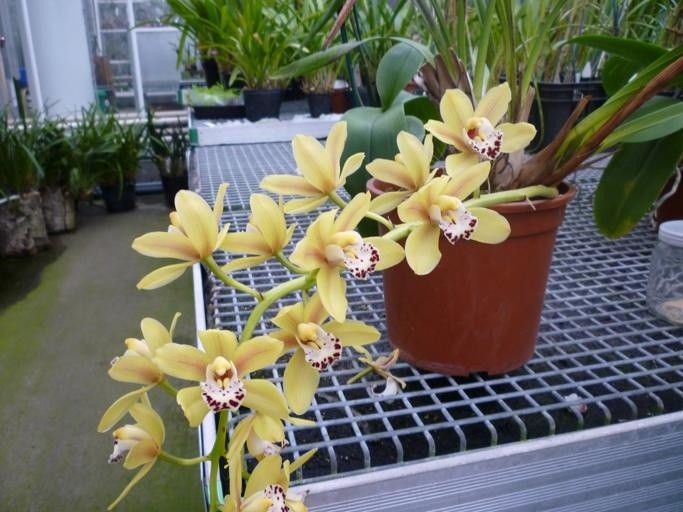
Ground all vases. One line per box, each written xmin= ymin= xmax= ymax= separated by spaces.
xmin=363 ymin=169 xmax=576 ymax=378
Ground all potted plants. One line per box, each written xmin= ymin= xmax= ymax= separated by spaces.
xmin=0 ymin=0 xmax=683 ymax=250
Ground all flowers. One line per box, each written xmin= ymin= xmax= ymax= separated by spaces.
xmin=97 ymin=78 xmax=564 ymax=511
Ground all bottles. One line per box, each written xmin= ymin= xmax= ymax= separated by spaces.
xmin=646 ymin=220 xmax=682 ymax=327
